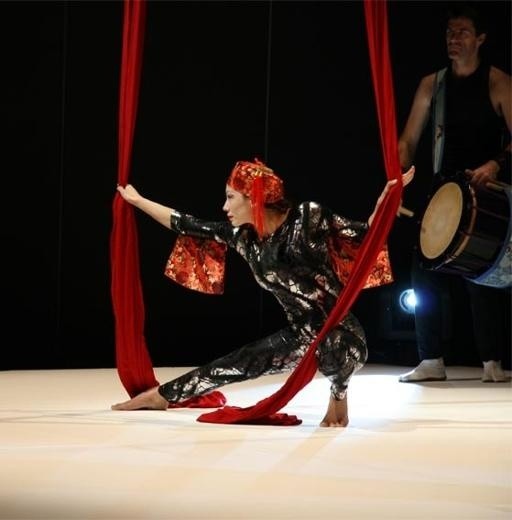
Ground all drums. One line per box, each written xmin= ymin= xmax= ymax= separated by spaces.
xmin=412 ymin=168 xmax=512 ymax=289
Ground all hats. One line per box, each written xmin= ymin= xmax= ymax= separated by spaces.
xmin=228 ymin=161 xmax=284 ymax=201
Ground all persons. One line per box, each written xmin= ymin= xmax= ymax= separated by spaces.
xmin=109 ymin=158 xmax=417 ymax=431
xmin=393 ymin=9 xmax=510 ymax=383
